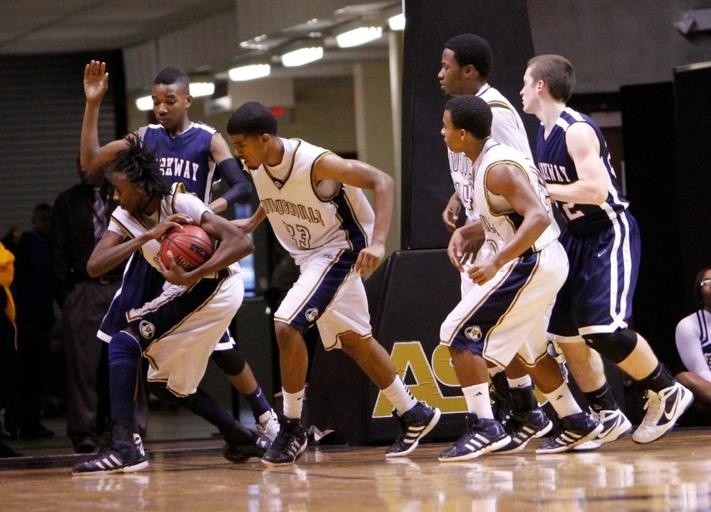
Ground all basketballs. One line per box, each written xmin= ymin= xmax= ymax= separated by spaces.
xmin=161 ymin=225 xmax=213 ymax=272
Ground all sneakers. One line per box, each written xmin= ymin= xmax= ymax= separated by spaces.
xmin=632 ymin=381 xmax=695 ymax=444
xmin=438 ymin=394 xmax=632 ymax=461
xmin=384 ymin=400 xmax=441 ymax=456
xmin=71 ymin=432 xmax=147 ymax=476
xmin=19 ymin=426 xmax=54 ymax=440
xmin=222 ymin=410 xmax=308 ymax=466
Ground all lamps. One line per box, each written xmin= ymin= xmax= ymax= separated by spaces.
xmin=278 ymin=37 xmax=324 ymax=69
xmin=230 ymin=59 xmax=271 ymax=83
xmin=334 ymin=19 xmax=382 ymax=50
xmin=387 ymin=13 xmax=406 ymax=33
xmin=135 ymin=92 xmax=156 ymax=113
xmin=186 ymin=76 xmax=217 ymax=98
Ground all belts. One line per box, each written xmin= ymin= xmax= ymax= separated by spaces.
xmin=78 ymin=274 xmax=122 ymax=284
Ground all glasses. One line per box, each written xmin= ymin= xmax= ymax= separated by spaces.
xmin=699 ymin=278 xmax=710 ymax=288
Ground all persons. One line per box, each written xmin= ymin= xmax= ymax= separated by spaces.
xmin=438 ymin=96 xmax=604 ymax=462
xmin=673 ymin=267 xmax=711 ymax=427
xmin=1 ymin=147 xmax=132 ymax=459
xmin=226 ymin=101 xmax=442 ymax=467
xmin=520 ymin=54 xmax=696 ymax=451
xmin=437 ymin=35 xmax=554 ymax=455
xmin=71 ymin=135 xmax=273 ymax=476
xmin=80 ymin=59 xmax=280 ymax=458
